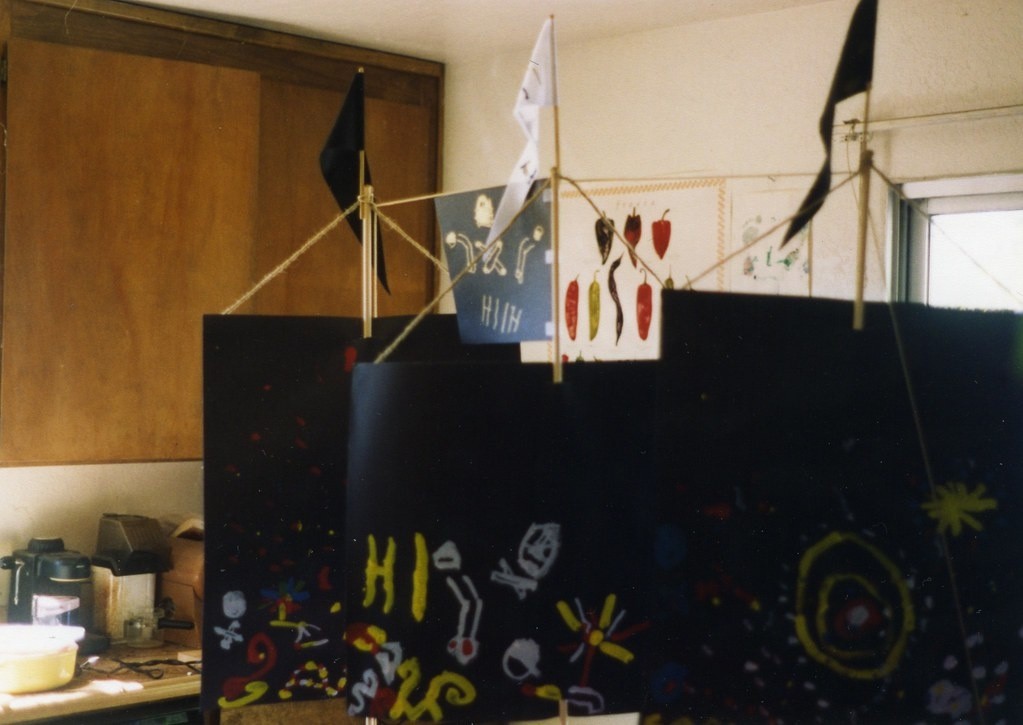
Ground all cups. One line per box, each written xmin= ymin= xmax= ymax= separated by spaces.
xmin=126 ymin=607 xmax=195 ymax=647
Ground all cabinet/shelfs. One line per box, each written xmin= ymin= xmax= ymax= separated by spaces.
xmin=0 ymin=2 xmax=446 ymax=469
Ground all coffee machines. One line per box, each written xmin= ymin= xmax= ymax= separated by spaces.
xmin=0 ymin=536 xmax=107 ymax=651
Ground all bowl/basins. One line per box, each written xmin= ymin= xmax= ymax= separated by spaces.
xmin=1 ymin=642 xmax=78 ymax=694
